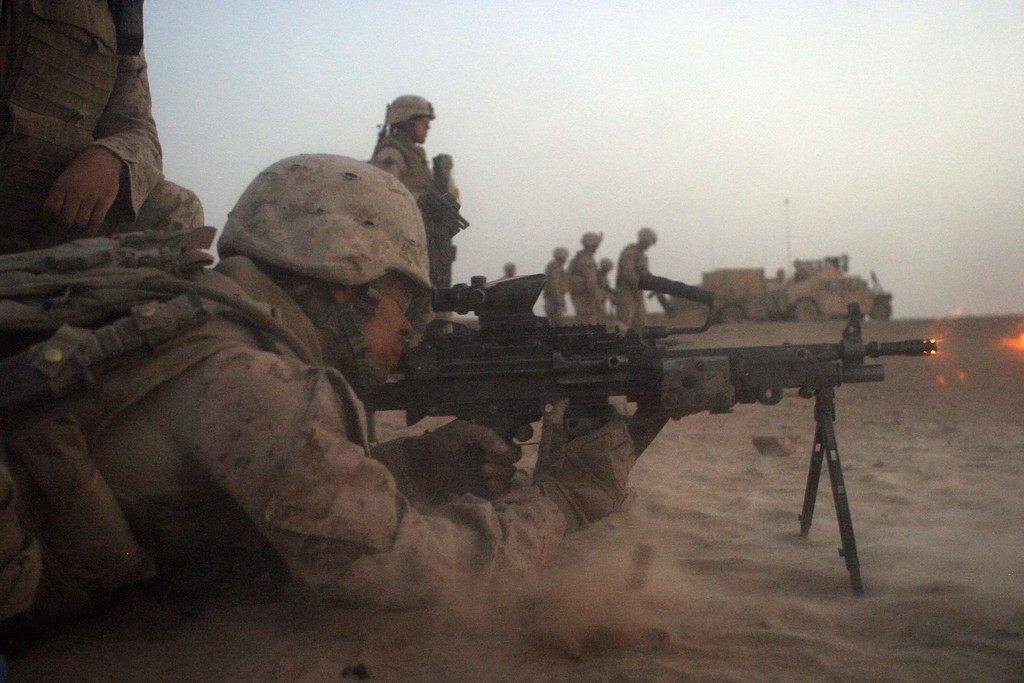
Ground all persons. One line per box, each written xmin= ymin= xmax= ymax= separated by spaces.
xmin=568 ymin=232 xmax=604 ymax=315
xmin=613 ymin=230 xmax=658 ymax=329
xmin=0 ymin=0 xmax=203 ymax=246
xmin=544 ymin=246 xmax=568 ymax=317
xmin=0 ymin=154 xmax=635 ymax=661
xmin=499 ymin=261 xmax=517 ymax=280
xmin=597 ymin=258 xmax=616 ymax=293
xmin=370 ymin=95 xmax=458 ymax=291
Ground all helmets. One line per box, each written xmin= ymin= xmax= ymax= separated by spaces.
xmin=387 ymin=95 xmax=436 ymax=125
xmin=216 ymin=153 xmax=433 ymax=292
xmin=553 ymin=247 xmax=569 ymax=259
xmin=638 ymin=227 xmax=658 ymax=244
xmin=598 ymin=257 xmax=614 ymax=267
xmin=580 ymin=232 xmax=604 ymax=243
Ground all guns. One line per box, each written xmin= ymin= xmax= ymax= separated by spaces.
xmin=415 ymin=185 xmax=470 ymax=230
xmin=355 ymin=274 xmax=937 ymax=597
xmin=638 ymin=265 xmax=675 ymax=314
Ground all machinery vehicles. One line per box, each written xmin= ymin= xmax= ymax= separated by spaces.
xmin=694 ymin=256 xmax=893 ymax=324
